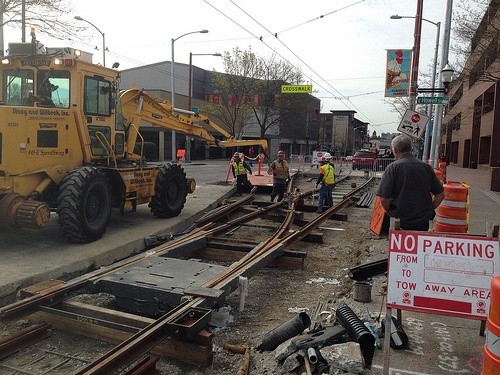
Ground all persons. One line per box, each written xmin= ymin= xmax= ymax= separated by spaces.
xmin=322 ymin=152 xmax=334 ymax=207
xmin=230 ymin=153 xmax=252 ymax=196
xmin=266 ymin=150 xmax=291 ymax=203
xmin=315 ymin=158 xmax=334 ymax=214
xmin=378 ymin=134 xmax=445 ymax=233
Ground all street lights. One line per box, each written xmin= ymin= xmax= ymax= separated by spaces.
xmin=390 ymin=15 xmax=441 ymax=164
xmin=74 ymin=16 xmax=107 ymax=68
xmin=170 ymin=29 xmax=222 ymax=164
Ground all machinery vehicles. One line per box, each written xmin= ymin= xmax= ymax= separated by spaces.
xmin=0 ymin=28 xmax=267 ymax=246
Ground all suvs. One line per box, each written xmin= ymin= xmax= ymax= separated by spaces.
xmin=352 ymin=150 xmax=376 ymax=172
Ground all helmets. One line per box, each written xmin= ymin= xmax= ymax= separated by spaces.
xmin=324 ymin=153 xmax=332 ymax=158
xmin=278 ymin=151 xmax=285 ymax=156
xmin=234 ymin=153 xmax=239 ymax=156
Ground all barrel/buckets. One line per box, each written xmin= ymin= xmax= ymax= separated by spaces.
xmin=354 ymin=283 xmax=373 ymax=303
